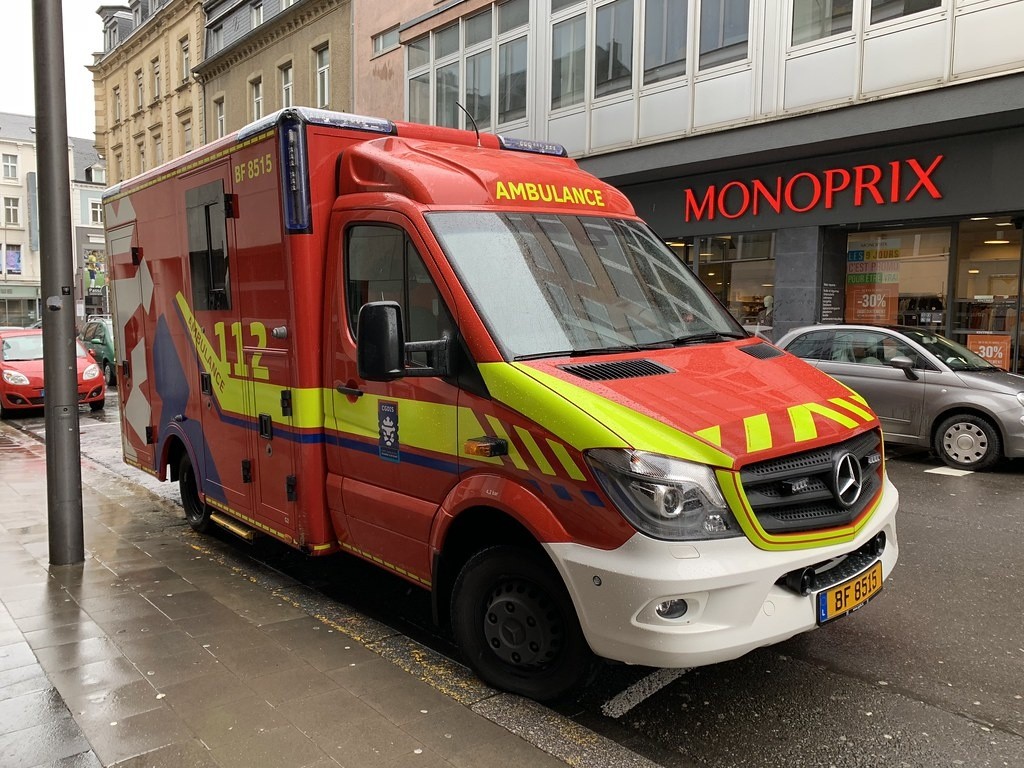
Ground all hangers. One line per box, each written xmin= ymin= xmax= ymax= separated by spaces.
xmin=967 ymin=298 xmax=1021 ymax=315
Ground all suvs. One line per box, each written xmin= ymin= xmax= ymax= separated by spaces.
xmin=79 ymin=317 xmax=117 ymax=386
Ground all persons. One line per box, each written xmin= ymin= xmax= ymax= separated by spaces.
xmin=756 ymin=295 xmax=773 ymax=326
xmin=87 ymin=250 xmax=98 ymax=292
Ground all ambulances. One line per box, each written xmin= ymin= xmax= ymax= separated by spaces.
xmin=101 ymin=106 xmax=900 ymax=708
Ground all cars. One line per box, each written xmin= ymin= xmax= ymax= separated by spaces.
xmin=0 ymin=329 xmax=105 ymax=420
xmin=774 ymin=322 xmax=1024 ymax=471
